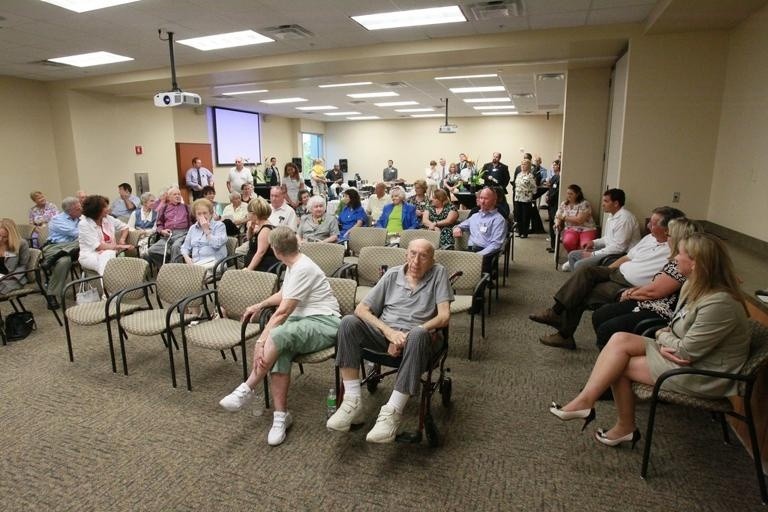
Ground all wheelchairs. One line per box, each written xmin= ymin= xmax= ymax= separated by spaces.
xmin=338 ymin=263 xmax=464 ymax=450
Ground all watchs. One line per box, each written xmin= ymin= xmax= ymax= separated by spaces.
xmin=255 ymin=339 xmax=265 ymax=345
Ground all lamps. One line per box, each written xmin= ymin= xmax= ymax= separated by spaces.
xmin=463 ymin=97 xmax=512 ymax=103
xmin=352 ymin=4 xmax=470 ymax=30
xmin=318 ymin=82 xmax=372 ymax=88
xmin=223 ymin=89 xmax=269 ymax=97
xmin=295 ymin=106 xmax=339 ymax=111
xmin=473 ymin=105 xmax=516 ymax=110
xmin=374 ymin=101 xmax=419 ymax=107
xmin=175 ymin=29 xmax=277 ymax=51
xmin=346 ymin=116 xmax=380 ymax=121
xmin=449 ymin=85 xmax=506 ymax=94
xmin=323 ymin=111 xmax=361 ymax=116
xmin=482 ymin=111 xmax=518 ymax=115
xmin=347 ymin=91 xmax=399 ymax=99
xmin=45 ymin=51 xmax=134 ymax=68
xmin=258 ymin=97 xmax=308 ymax=105
xmin=394 ymin=108 xmax=435 ymax=113
xmin=433 ymin=74 xmax=499 ymax=80
xmin=410 ymin=114 xmax=445 ymax=118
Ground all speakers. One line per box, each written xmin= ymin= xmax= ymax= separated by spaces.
xmin=292 ymin=158 xmax=302 ymax=173
xmin=339 ymin=159 xmax=348 ymax=174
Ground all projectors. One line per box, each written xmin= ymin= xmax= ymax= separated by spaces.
xmin=440 ymin=126 xmax=459 ymax=133
xmin=153 ymin=93 xmax=202 ymax=108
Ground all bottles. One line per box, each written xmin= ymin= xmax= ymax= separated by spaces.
xmin=327 ymin=388 xmax=336 ymax=416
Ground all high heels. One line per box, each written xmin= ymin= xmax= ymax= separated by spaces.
xmin=548 ymin=402 xmax=595 ymax=431
xmin=188 ymin=309 xmax=204 ymax=328
xmin=596 ymin=427 xmax=641 ymax=450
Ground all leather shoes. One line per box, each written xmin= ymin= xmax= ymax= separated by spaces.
xmin=47 ymin=295 xmax=59 ymax=309
xmin=528 ymin=308 xmax=561 ymax=329
xmin=539 ymin=331 xmax=575 ymax=349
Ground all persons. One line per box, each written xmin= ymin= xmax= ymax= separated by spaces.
xmin=226 ymin=157 xmax=254 ymax=193
xmin=201 ymin=185 xmax=222 ymax=222
xmin=567 ymin=187 xmax=642 ymax=274
xmin=296 ymin=189 xmax=312 ymax=228
xmin=147 ymin=186 xmax=192 ymax=263
xmin=456 ymin=152 xmax=467 ymax=174
xmin=366 ymin=183 xmax=393 ymax=225
xmin=553 ymin=184 xmax=597 ymax=272
xmin=180 ymin=198 xmax=227 ymax=329
xmin=549 ymin=232 xmax=753 ymax=451
xmin=580 ymin=216 xmax=705 ymax=402
xmin=263 ymin=156 xmax=281 ymax=186
xmin=29 ymin=190 xmax=59 ymax=247
xmin=443 ymin=163 xmax=462 ymax=205
xmin=529 ymin=205 xmax=686 ymax=350
xmin=512 ymin=152 xmax=541 ymax=222
xmin=374 ymin=186 xmax=417 ymax=247
xmin=460 ymin=161 xmax=476 ymax=191
xmin=235 ymin=185 xmax=298 ymax=269
xmin=325 ymin=238 xmax=455 ymax=445
xmin=218 ymin=225 xmax=343 ymax=446
xmin=326 ymin=162 xmax=344 ymax=200
xmin=296 ymin=195 xmax=341 ymax=246
xmin=422 ymin=189 xmax=459 ymax=250
xmin=77 ymin=194 xmax=135 ymax=302
xmin=425 ymin=159 xmax=440 ymax=190
xmin=241 ymin=183 xmax=254 ymax=204
xmin=452 ymin=186 xmax=508 ymax=314
xmin=513 ymin=159 xmax=538 ymax=238
xmin=407 ymin=179 xmax=430 ymax=229
xmin=533 ymin=155 xmax=547 ymax=184
xmin=479 ymin=152 xmax=510 ymax=194
xmin=242 ymin=198 xmax=286 ymax=272
xmin=77 ymin=190 xmax=86 ymax=204
xmin=221 ymin=191 xmax=248 ymax=235
xmin=282 ymin=162 xmax=305 ymax=210
xmin=107 ymin=183 xmax=141 ymax=218
xmin=126 ymin=191 xmax=157 ymax=259
xmin=185 ymin=157 xmax=216 ymax=201
xmin=337 ymin=188 xmax=369 ymax=256
xmin=382 ymin=159 xmax=398 ymax=181
xmin=438 ymin=156 xmax=450 ymax=189
xmin=546 ymin=159 xmax=561 ymax=253
xmin=0 ymin=217 xmax=29 ymax=296
xmin=43 ymin=196 xmax=82 ymax=310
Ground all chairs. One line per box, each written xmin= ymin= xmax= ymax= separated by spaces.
xmin=555 ymin=221 xmax=601 ymax=270
xmin=339 ymin=246 xmax=408 ymax=307
xmin=506 ymin=214 xmax=517 ymax=261
xmin=334 ymin=227 xmax=390 ymax=267
xmin=27 ymin=227 xmax=48 ymax=249
xmin=116 ymin=262 xmax=207 ymax=389
xmin=257 ymin=276 xmax=366 ymax=408
xmin=71 ymin=231 xmax=154 ymax=300
xmin=38 ymin=239 xmax=88 ymax=300
xmin=180 ymin=270 xmax=278 ymax=391
xmin=415 ymin=209 xmax=471 ymax=230
xmin=220 ymin=255 xmax=284 ymax=278
xmin=433 ymin=250 xmax=489 ymax=360
xmin=443 ymin=226 xmax=513 ymax=299
xmin=61 ymin=258 xmax=168 ymax=374
xmin=0 ymin=248 xmax=63 ymax=327
xmin=632 ymin=287 xmax=768 ymax=503
xmin=171 ymin=236 xmax=239 ymax=301
xmin=16 ymin=224 xmax=35 ymax=247
xmin=387 ymin=230 xmax=444 ymax=250
xmin=277 ymin=242 xmax=353 ymax=294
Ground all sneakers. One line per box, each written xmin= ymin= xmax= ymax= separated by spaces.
xmin=366 ymin=404 xmax=403 ymax=444
xmin=218 ymin=382 xmax=256 ymax=413
xmin=325 ymin=394 xmax=364 ymax=433
xmin=266 ymin=410 xmax=293 ymax=445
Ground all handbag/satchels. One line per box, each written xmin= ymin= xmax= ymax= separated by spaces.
xmin=76 ymin=278 xmax=100 ymax=305
xmin=193 ymin=257 xmax=216 ymax=270
xmin=4 ymin=311 xmax=37 ymax=342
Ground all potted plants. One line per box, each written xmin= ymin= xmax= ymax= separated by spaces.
xmin=468 ymin=168 xmax=487 ymax=194
